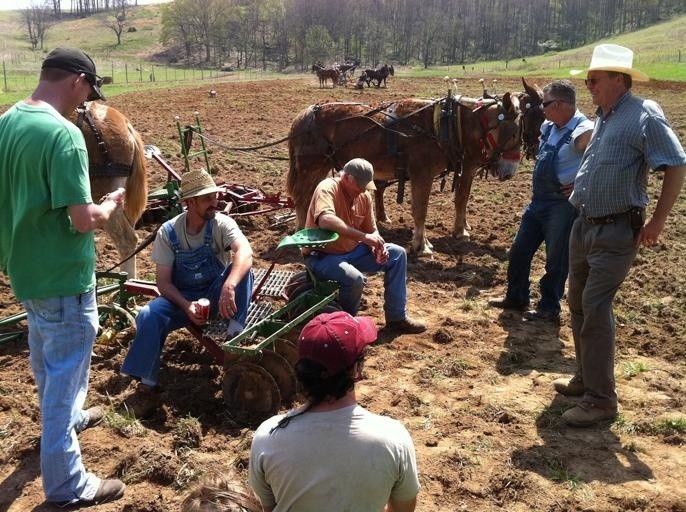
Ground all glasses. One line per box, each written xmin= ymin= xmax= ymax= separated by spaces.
xmin=79 ymin=70 xmax=104 ymax=88
xmin=585 ymin=75 xmax=609 ymax=86
xmin=543 ymin=99 xmax=566 ymax=108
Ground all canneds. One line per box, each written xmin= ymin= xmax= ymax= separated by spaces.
xmin=196 ymin=298 xmax=211 ymax=323
xmin=375 ymin=248 xmax=385 ymax=264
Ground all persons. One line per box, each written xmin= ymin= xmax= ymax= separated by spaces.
xmin=0 ymin=46 xmax=128 ymax=509
xmin=248 ymin=309 xmax=422 ymax=512
xmin=180 ymin=466 xmax=264 ymax=512
xmin=302 ymin=157 xmax=428 ymax=334
xmin=554 ymin=44 xmax=686 ymax=427
xmin=488 ymin=79 xmax=595 ymax=322
xmin=120 ymin=169 xmax=253 ymax=421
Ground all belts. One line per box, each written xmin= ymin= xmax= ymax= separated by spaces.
xmin=587 ymin=211 xmax=630 ymax=226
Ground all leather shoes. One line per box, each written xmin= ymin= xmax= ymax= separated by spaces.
xmin=58 ymin=479 xmax=126 ymax=508
xmin=82 ymin=406 xmax=104 ymax=426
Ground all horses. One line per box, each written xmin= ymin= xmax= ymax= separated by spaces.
xmin=369 ymin=75 xmax=547 ymax=228
xmin=286 ymin=89 xmax=526 ymax=255
xmin=359 ymin=64 xmax=397 ymax=88
xmin=0 ymin=102 xmax=153 ymax=283
xmin=310 ymin=59 xmax=356 ymax=88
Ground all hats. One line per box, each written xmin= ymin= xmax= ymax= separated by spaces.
xmin=570 ymin=43 xmax=650 ymax=82
xmin=296 ymin=310 xmax=378 ymax=377
xmin=177 ymin=168 xmax=227 ymax=204
xmin=344 ymin=158 xmax=377 ymax=191
xmin=41 ymin=46 xmax=106 ymax=102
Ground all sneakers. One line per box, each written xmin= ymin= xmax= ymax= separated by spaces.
xmin=488 ymin=297 xmax=530 ymax=308
xmin=562 ymin=402 xmax=619 ymax=427
xmin=386 ymin=317 xmax=426 ymax=334
xmin=122 ymin=383 xmax=158 ymax=418
xmin=554 ymin=380 xmax=584 ymax=396
xmin=524 ymin=310 xmax=561 ymax=320
